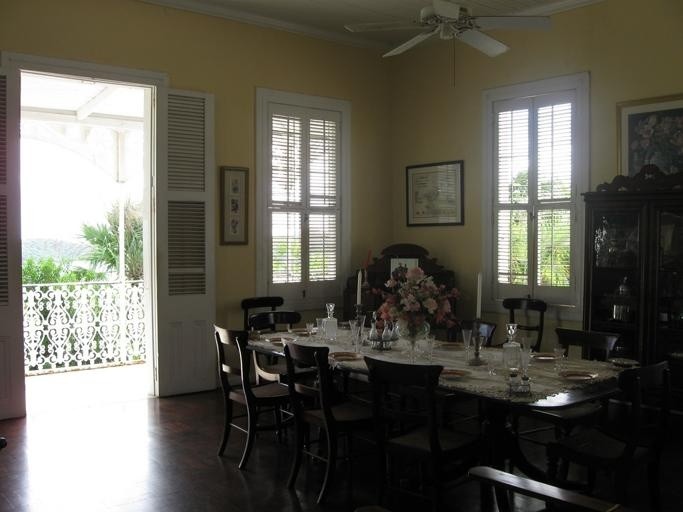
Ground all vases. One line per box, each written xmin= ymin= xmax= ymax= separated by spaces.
xmin=395 ymin=317 xmax=430 ymax=364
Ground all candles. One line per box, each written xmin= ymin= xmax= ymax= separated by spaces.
xmin=476 ymin=273 xmax=482 ymax=320
xmin=357 ymin=268 xmax=362 ymax=305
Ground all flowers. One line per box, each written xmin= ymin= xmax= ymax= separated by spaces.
xmin=371 ymin=265 xmax=460 ymax=327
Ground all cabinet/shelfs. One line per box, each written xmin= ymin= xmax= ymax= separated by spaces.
xmin=581 ymin=164 xmax=683 ymax=362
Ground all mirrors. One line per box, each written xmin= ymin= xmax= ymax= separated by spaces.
xmin=391 ymin=258 xmax=418 ymax=280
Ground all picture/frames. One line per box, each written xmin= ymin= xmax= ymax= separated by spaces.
xmin=616 ymin=93 xmax=683 ymax=178
xmin=405 ymin=160 xmax=464 ymax=227
xmin=219 ymin=166 xmax=249 ymax=246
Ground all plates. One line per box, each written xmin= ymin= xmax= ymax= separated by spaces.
xmin=534 ymin=354 xmax=564 ymax=360
xmin=608 ymin=358 xmax=638 ymax=367
xmin=560 ymin=370 xmax=598 ymax=382
xmin=440 ymin=369 xmax=472 ymax=380
xmin=330 ymin=351 xmax=358 ymax=361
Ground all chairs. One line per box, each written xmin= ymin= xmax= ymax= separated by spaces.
xmin=241 ymin=296 xmax=285 ymax=332
xmin=554 ymin=326 xmax=620 ymax=361
xmin=284 ymin=341 xmax=377 ymax=509
xmin=446 ymin=320 xmax=496 ymax=348
xmin=248 ymin=311 xmax=317 ymax=420
xmin=248 ymin=310 xmax=302 ymax=334
xmin=363 ymin=356 xmax=513 ymax=512
xmin=503 ymin=298 xmax=547 ymax=353
xmin=213 ymin=323 xmax=305 ymax=470
xmin=610 ymin=361 xmax=671 ymax=458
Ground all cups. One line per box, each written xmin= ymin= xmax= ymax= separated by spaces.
xmin=350 ymin=320 xmax=361 ymax=352
xmin=463 ymin=330 xmax=472 ymax=360
xmin=521 ymin=336 xmax=532 ymax=377
xmin=427 ymin=335 xmax=435 ymax=365
xmin=316 ymin=318 xmax=323 ymax=332
xmin=488 ymin=355 xmax=496 ymax=376
xmin=306 ymin=322 xmax=313 ymax=342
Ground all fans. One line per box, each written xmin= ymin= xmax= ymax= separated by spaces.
xmin=343 ymin=0 xmax=550 ymax=59
